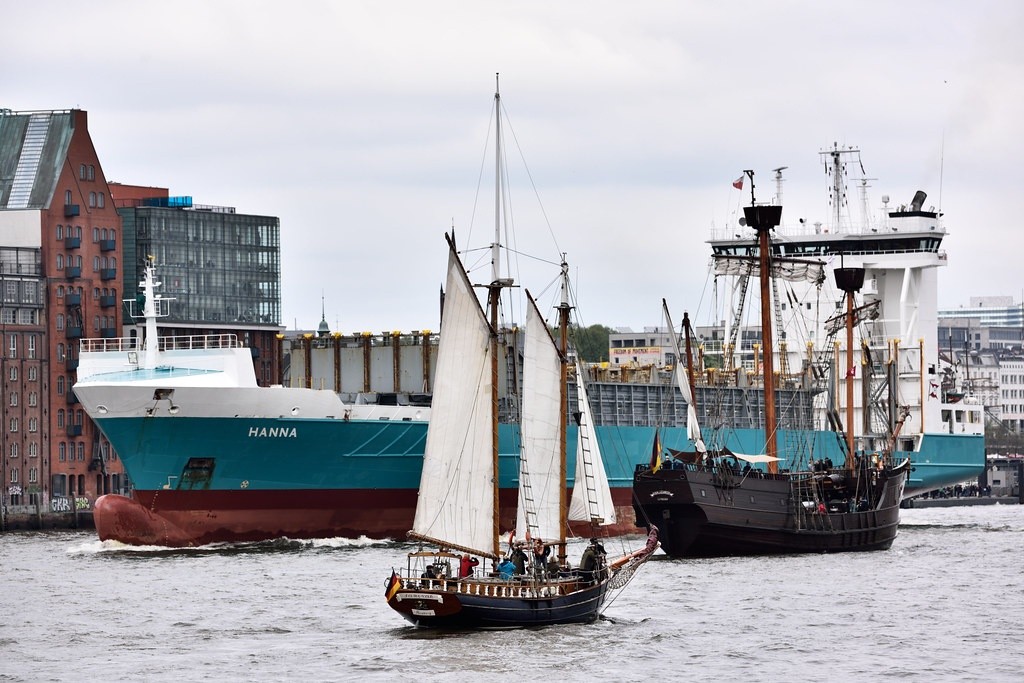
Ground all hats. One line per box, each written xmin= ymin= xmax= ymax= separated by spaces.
xmin=516 ymin=542 xmax=524 ymax=547
xmin=426 ymin=565 xmax=434 ymax=569
xmin=503 ymin=555 xmax=510 ymax=561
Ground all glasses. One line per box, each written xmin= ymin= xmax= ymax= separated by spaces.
xmin=537 ymin=542 xmax=539 ymax=544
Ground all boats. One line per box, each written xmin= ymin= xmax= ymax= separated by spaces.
xmin=72 ymin=144 xmax=987 ymax=547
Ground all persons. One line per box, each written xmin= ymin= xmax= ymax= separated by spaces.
xmin=533 ymin=538 xmax=560 ymax=578
xmin=510 ymin=547 xmax=527 ymax=575
xmin=497 ymin=556 xmax=515 ymax=580
xmin=460 ymin=554 xmax=479 ymax=578
xmin=580 ymin=538 xmax=606 ymax=588
xmin=421 ymin=565 xmax=440 ymax=587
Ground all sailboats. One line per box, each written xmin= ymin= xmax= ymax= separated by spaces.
xmin=384 ymin=73 xmax=659 ymax=634
xmin=634 ymin=163 xmax=909 ymax=558
